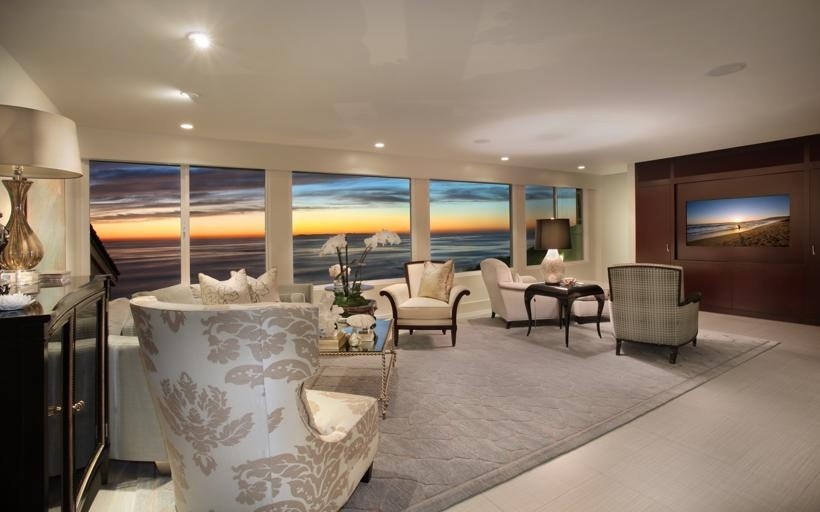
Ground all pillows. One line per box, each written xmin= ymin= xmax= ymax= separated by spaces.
xmin=512 ymin=273 xmax=524 ymax=283
xmin=419 ymin=260 xmax=454 ymax=304
xmin=133 ymin=284 xmax=198 ymax=305
xmin=198 ymin=269 xmax=250 ymax=303
xmin=229 ymin=266 xmax=280 ymax=302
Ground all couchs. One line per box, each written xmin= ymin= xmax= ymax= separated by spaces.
xmin=97 ymin=282 xmax=313 ymax=476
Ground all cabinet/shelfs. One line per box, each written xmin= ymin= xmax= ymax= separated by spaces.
xmin=635 ymin=133 xmax=820 ymax=325
xmin=0 ymin=274 xmax=111 ymax=512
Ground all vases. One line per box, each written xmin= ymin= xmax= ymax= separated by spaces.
xmin=342 ymin=299 xmax=377 ymax=319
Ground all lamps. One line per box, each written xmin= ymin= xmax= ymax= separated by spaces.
xmin=534 ymin=218 xmax=572 ymax=285
xmin=1 ymin=105 xmax=83 ymax=293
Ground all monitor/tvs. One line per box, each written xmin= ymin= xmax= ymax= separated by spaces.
xmin=686 ymin=194 xmax=792 ymax=248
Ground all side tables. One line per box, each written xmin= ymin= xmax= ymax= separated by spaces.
xmin=524 ymin=281 xmax=605 ymax=347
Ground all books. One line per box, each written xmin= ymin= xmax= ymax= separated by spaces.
xmin=318 ymin=330 xmax=351 ymax=352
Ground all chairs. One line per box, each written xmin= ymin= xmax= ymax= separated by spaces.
xmin=607 ymin=264 xmax=700 ymax=364
xmin=129 ymin=296 xmax=379 ymax=512
xmin=379 ymin=260 xmax=470 ymax=346
xmin=480 ymin=258 xmax=571 ymax=330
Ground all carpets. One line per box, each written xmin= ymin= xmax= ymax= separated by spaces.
xmin=134 ymin=310 xmax=781 ymax=512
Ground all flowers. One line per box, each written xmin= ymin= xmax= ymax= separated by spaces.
xmin=318 ymin=229 xmax=401 ymax=307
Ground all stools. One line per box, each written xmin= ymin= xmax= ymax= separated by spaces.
xmin=571 ymin=289 xmax=611 ymax=322
